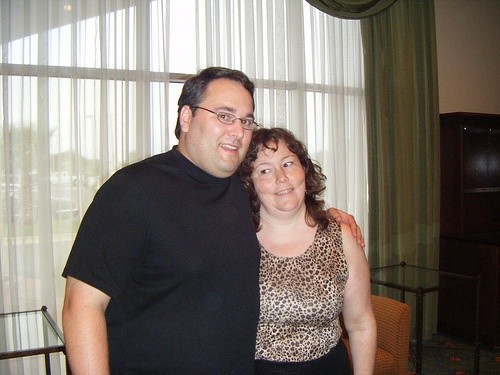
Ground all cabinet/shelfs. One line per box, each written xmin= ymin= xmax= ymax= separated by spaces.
xmin=439 ymin=112 xmax=500 ymax=350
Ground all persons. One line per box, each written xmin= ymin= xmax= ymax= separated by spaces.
xmin=61 ymin=67 xmax=366 ymax=375
xmin=239 ymin=128 xmax=377 ymax=375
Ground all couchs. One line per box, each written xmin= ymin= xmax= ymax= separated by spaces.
xmin=341 ymin=296 xmax=412 ymax=375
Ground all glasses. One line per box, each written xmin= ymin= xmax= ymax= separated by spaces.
xmin=191 ymin=106 xmax=259 ymax=130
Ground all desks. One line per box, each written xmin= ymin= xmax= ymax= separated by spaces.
xmin=0 ymin=306 xmax=72 ymax=375
xmin=370 ymin=261 xmax=481 ymax=375
